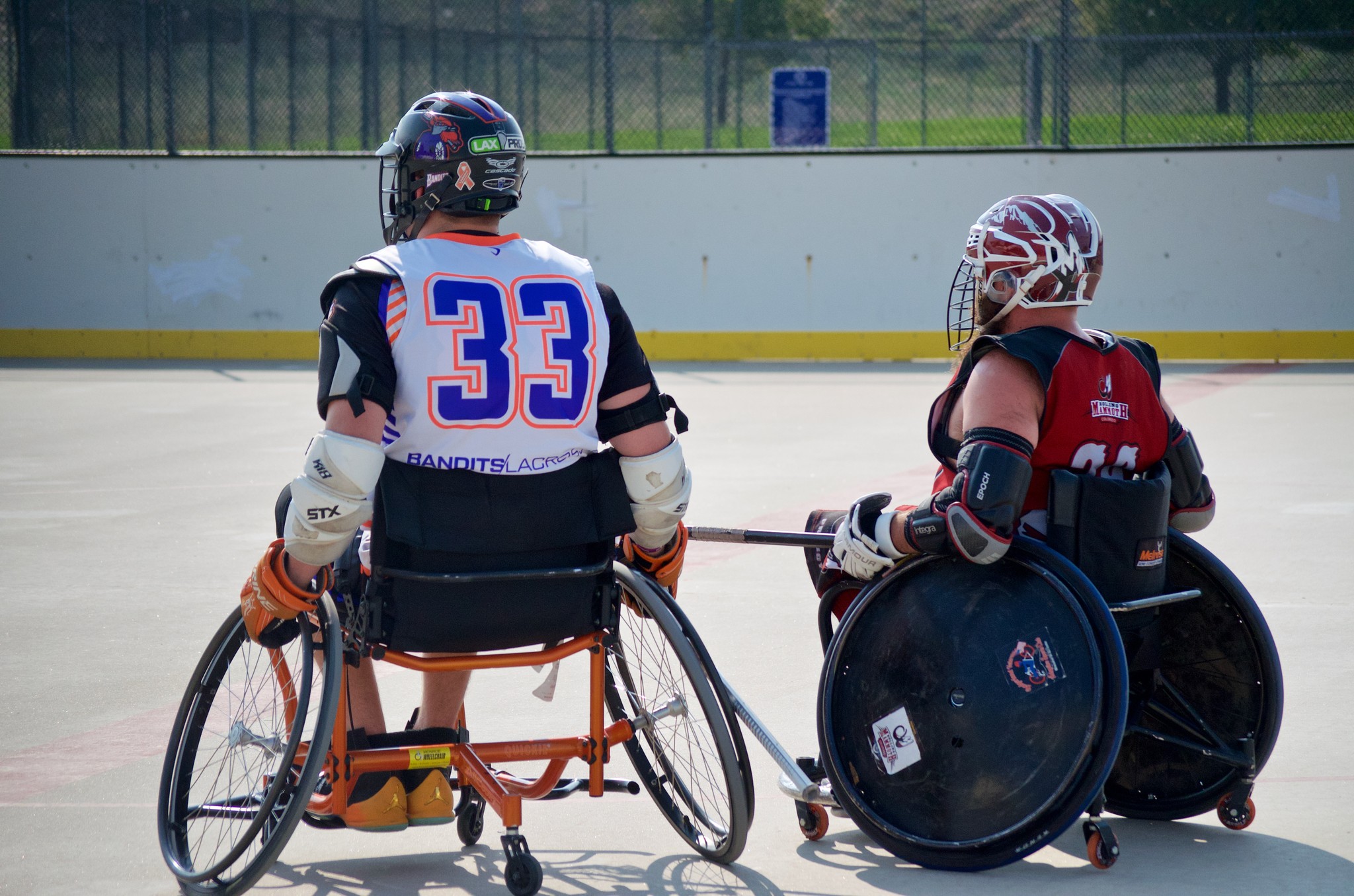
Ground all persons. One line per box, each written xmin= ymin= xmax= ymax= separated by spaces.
xmin=804 ymin=193 xmax=1215 ymax=629
xmin=247 ymin=90 xmax=693 ymax=831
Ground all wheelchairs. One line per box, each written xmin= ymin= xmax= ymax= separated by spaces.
xmin=793 ymin=459 xmax=1284 ymax=873
xmin=158 ymin=487 xmax=754 ymax=896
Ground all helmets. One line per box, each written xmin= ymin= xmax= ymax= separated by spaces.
xmin=373 ymin=92 xmax=528 ymax=217
xmin=965 ymin=193 xmax=1106 ymax=309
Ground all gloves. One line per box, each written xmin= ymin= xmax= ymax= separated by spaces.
xmin=241 ymin=536 xmax=335 ymax=649
xmin=819 ymin=491 xmax=901 ymax=595
xmin=617 ymin=518 xmax=689 ymax=621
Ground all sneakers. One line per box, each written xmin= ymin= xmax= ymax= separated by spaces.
xmin=403 ymin=708 xmax=457 ymax=824
xmin=306 ymin=727 xmax=408 ymax=832
